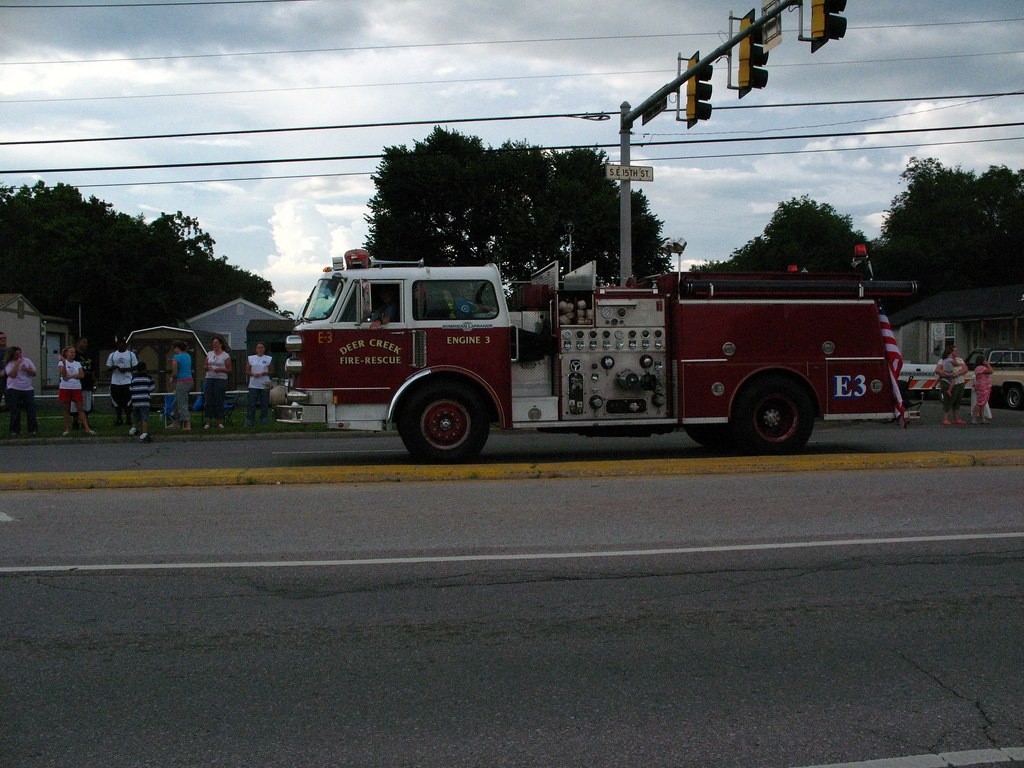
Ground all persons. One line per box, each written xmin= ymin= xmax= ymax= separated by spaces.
xmin=57 ymin=337 xmax=97 ymax=436
xmin=127 ymin=362 xmax=156 ymax=441
xmin=0 ymin=331 xmax=38 ymax=438
xmin=322 ymin=281 xmax=356 ymax=322
xmin=932 ymin=345 xmax=967 ymax=426
xmin=454 ymin=282 xmax=490 ymax=320
xmin=368 ymin=289 xmax=396 ymax=329
xmin=970 ymin=355 xmax=993 ymax=424
xmin=244 ymin=341 xmax=274 ymax=427
xmin=169 ymin=340 xmax=194 ymax=431
xmin=203 ymin=336 xmax=232 ymax=430
xmin=105 ymin=338 xmax=139 ymax=426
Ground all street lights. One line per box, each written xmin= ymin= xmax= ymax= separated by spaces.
xmin=660 ymin=238 xmax=687 ymax=284
xmin=564 ymin=222 xmax=575 ymax=271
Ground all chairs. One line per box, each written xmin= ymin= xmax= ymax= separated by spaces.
xmin=162 ymin=379 xmax=238 ymax=429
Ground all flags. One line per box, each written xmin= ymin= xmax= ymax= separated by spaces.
xmin=866 ymin=255 xmax=906 ymax=429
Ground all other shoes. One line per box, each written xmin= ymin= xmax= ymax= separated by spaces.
xmin=140 ymin=433 xmax=148 ymax=439
xmin=971 ymin=418 xmax=978 ymax=424
xmin=83 ymin=429 xmax=97 ymax=435
xmin=216 ymin=423 xmax=224 ymax=429
xmin=129 ymin=428 xmax=136 ymax=434
xmin=166 ymin=424 xmax=179 ymax=430
xmin=943 ymin=419 xmax=951 ymax=425
xmin=62 ymin=432 xmax=71 ymax=437
xmin=953 ymin=418 xmax=967 ymax=424
xmin=947 ymin=390 xmax=951 ymax=396
xmin=204 ymin=424 xmax=210 ymax=429
xmin=181 ymin=427 xmax=192 ymax=431
xmin=932 ymin=384 xmax=936 ymax=390
xmin=113 ymin=418 xmax=123 ymax=426
xmin=981 ymin=420 xmax=990 ymax=425
xmin=127 ymin=419 xmax=133 ymax=424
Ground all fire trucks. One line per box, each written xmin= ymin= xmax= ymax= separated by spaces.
xmin=269 ymin=244 xmax=922 ymax=458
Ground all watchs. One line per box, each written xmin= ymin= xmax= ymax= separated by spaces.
xmin=378 ymin=316 xmax=384 ymax=325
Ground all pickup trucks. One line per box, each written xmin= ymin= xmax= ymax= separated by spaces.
xmin=939 ymin=346 xmax=1024 ymax=410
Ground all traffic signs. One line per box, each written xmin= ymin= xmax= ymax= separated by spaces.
xmin=605 ymin=163 xmax=654 ymax=181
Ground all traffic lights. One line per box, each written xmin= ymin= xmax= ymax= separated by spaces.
xmin=686 ymin=52 xmax=712 ymax=126
xmin=738 ymin=9 xmax=768 ymax=98
xmin=812 ymin=1 xmax=847 ymax=53
